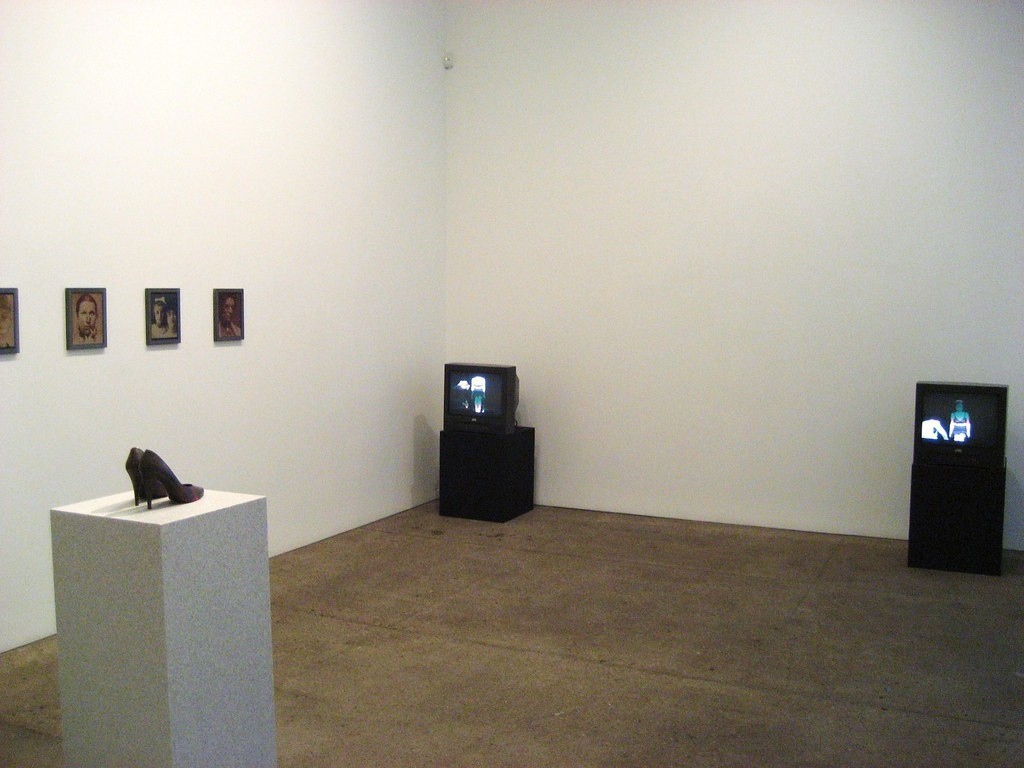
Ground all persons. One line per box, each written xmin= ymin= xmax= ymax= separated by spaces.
xmin=74 ymin=294 xmax=103 ymax=345
xmin=470 ymin=377 xmax=486 ymax=413
xmin=219 ymin=296 xmax=241 ymax=336
xmin=152 ymin=297 xmax=177 ymax=339
xmin=921 ymin=416 xmax=948 ymax=440
xmin=949 ymin=399 xmax=970 ymax=442
xmin=450 ymin=380 xmax=470 ymax=410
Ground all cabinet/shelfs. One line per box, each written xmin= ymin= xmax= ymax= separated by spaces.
xmin=908 ymin=457 xmax=1008 ymax=576
xmin=438 ymin=427 xmax=536 ymax=523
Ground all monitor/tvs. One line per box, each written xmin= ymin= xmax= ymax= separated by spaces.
xmin=913 ymin=382 xmax=1008 ymax=469
xmin=443 ymin=363 xmax=519 ymax=434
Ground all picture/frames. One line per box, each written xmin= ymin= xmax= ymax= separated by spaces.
xmin=65 ymin=288 xmax=107 ymax=350
xmin=0 ymin=288 xmax=19 ymax=355
xmin=213 ymin=289 xmax=245 ymax=341
xmin=145 ymin=288 xmax=181 ymax=345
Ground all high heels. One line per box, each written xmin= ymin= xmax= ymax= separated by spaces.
xmin=126 ymin=447 xmax=204 ymax=509
